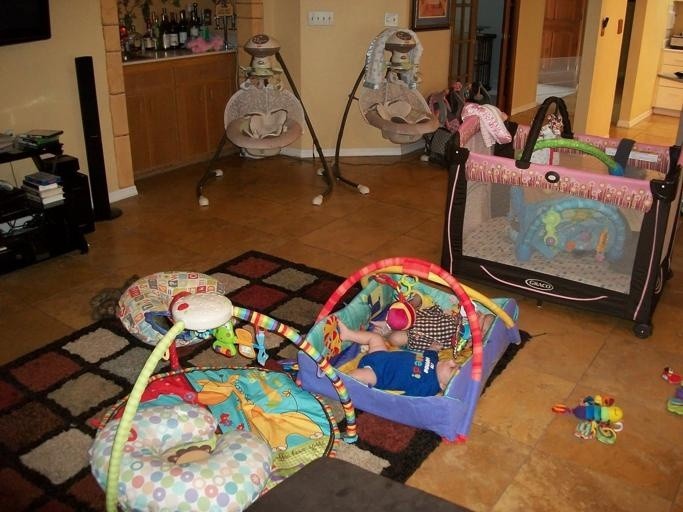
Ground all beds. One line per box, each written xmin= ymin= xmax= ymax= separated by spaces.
xmin=440 ymin=95 xmax=683 ymax=339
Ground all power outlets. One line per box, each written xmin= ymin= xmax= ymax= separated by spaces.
xmin=307 ymin=11 xmax=334 ymax=26
xmin=384 ymin=13 xmax=399 ymax=27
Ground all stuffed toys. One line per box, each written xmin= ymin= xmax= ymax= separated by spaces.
xmin=550 ymin=393 xmax=626 ymax=446
xmin=235 ymin=328 xmax=257 ymax=359
xmin=660 ymin=366 xmax=683 ymax=416
xmin=211 ymin=320 xmax=237 ymax=357
xmin=527 ymin=113 xmax=562 ymax=164
xmin=454 ymin=301 xmax=478 ymax=353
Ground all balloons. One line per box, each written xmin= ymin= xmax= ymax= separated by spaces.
xmin=387 ymin=301 xmax=416 ymax=331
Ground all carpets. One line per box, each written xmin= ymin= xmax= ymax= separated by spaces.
xmin=0 ymin=248 xmax=532 ymax=512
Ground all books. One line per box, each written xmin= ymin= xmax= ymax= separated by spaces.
xmin=21 ymin=171 xmax=66 ymax=210
xmin=14 ymin=129 xmax=66 ymax=153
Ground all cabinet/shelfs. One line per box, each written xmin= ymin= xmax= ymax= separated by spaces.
xmin=173 ymin=52 xmax=241 ymax=167
xmin=121 ymin=61 xmax=181 ymax=180
xmin=0 ymin=140 xmax=96 ymax=279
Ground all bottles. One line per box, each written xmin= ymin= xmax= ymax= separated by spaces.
xmin=128 ymin=25 xmax=142 ymax=57
xmin=189 ymin=10 xmax=198 ymax=38
xmin=169 ymin=12 xmax=178 ymax=49
xmin=161 ymin=14 xmax=169 ymax=50
xmin=143 ymin=12 xmax=160 ymax=51
xmin=179 ymin=11 xmax=188 ymax=45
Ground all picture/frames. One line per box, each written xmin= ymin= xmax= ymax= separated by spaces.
xmin=412 ymin=0 xmax=451 ymax=30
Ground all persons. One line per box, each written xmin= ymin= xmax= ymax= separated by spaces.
xmin=374 ymin=289 xmax=494 ymax=353
xmin=336 ymin=318 xmax=472 ymax=396
